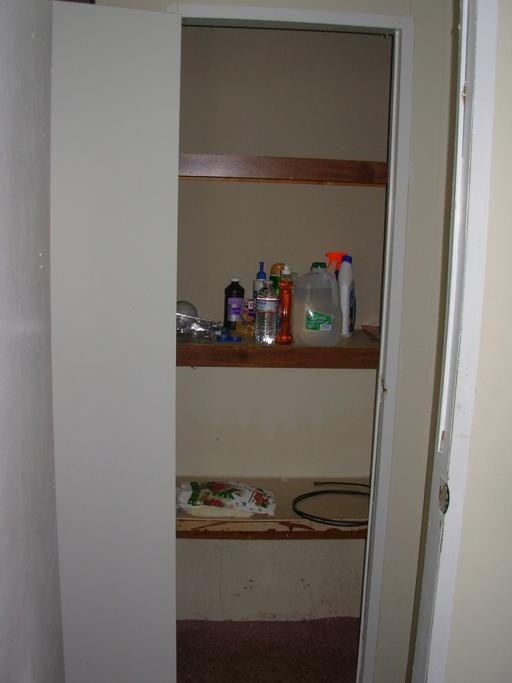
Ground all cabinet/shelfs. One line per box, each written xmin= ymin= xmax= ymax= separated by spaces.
xmin=171 ymin=150 xmax=389 ymax=550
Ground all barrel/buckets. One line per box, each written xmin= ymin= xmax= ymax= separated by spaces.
xmin=289 ymin=261 xmax=343 ymax=347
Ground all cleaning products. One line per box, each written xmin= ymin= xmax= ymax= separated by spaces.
xmin=251 ymin=261 xmax=266 ymax=298
xmin=277 ymin=263 xmax=293 ymax=345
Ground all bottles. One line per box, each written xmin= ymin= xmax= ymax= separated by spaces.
xmin=251 ymin=260 xmax=295 ymax=346
xmin=224 ymin=277 xmax=244 ymax=329
